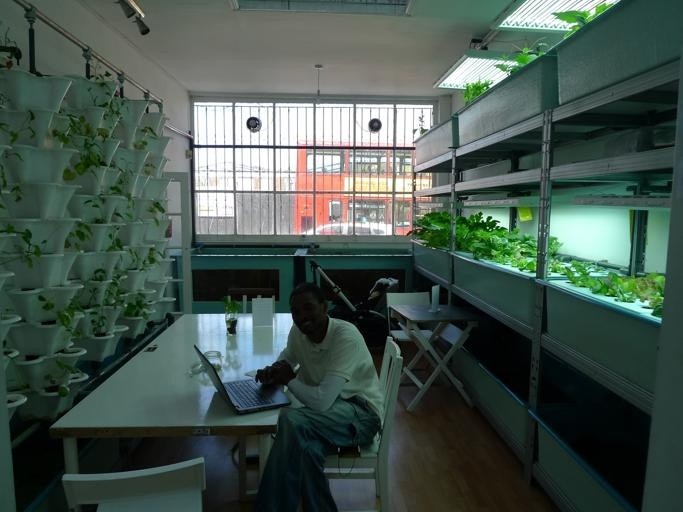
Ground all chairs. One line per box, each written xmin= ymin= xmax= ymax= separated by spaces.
xmin=386 ymin=292 xmax=433 ymax=387
xmin=62 ymin=456 xmax=206 ymax=511
xmin=322 ymin=337 xmax=405 ymax=512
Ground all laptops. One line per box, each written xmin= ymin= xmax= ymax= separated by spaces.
xmin=192 ymin=344 xmax=291 ymax=414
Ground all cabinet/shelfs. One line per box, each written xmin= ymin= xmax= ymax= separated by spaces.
xmin=411 ymin=62 xmax=682 ymax=512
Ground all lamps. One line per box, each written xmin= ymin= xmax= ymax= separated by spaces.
xmin=314 ymin=64 xmax=324 ymax=104
xmin=490 ymin=1 xmax=619 ymax=33
xmin=432 ymin=50 xmax=518 ymax=92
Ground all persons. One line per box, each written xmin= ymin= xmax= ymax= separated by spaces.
xmin=255 ymin=283 xmax=383 ymax=512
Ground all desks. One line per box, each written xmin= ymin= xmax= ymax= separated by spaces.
xmin=49 ymin=312 xmax=293 ymax=495
xmin=389 ymin=305 xmax=478 ymax=412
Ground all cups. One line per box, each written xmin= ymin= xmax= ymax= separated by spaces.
xmin=201 ymin=351 xmax=224 ymax=374
